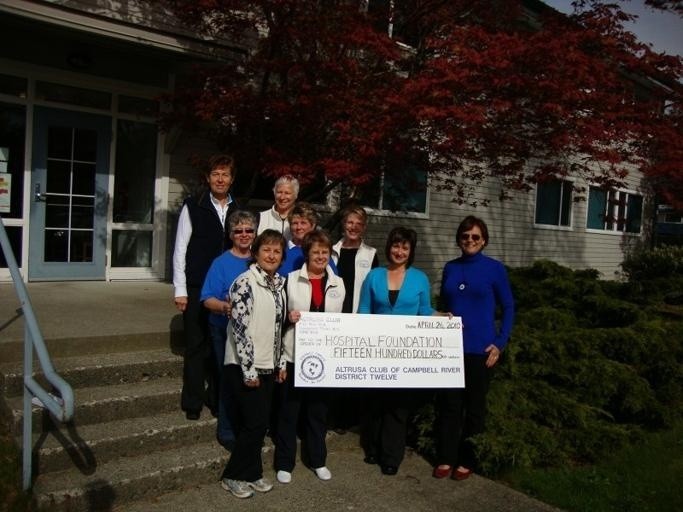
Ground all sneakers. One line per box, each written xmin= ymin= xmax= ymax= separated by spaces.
xmin=314 ymin=466 xmax=332 ymax=481
xmin=276 ymin=469 xmax=293 ymax=484
xmin=365 ymin=455 xmax=399 ymax=475
xmin=250 ymin=478 xmax=274 ymax=495
xmin=220 ymin=475 xmax=255 ymax=500
xmin=185 ymin=407 xmax=221 ymax=422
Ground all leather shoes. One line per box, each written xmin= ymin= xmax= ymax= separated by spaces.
xmin=432 ymin=464 xmax=471 ymax=481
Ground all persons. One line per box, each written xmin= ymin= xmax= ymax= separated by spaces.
xmin=170 ymin=153 xmax=242 ymax=422
xmin=216 ymin=229 xmax=288 ymax=500
xmin=431 ymin=214 xmax=517 ymax=481
xmin=273 ymin=200 xmax=339 ymax=280
xmin=270 ymin=230 xmax=347 ymax=487
xmin=354 ymin=227 xmax=465 ymax=476
xmin=253 ymin=174 xmax=323 ymax=250
xmin=198 ymin=208 xmax=266 ymax=452
xmin=327 ymin=203 xmax=380 ymax=430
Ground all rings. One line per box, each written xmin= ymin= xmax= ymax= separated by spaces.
xmin=494 ymin=355 xmax=496 ymax=361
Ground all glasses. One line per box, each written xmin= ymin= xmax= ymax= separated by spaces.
xmin=458 ymin=233 xmax=483 ymax=241
xmin=229 ymin=228 xmax=255 ymax=234
